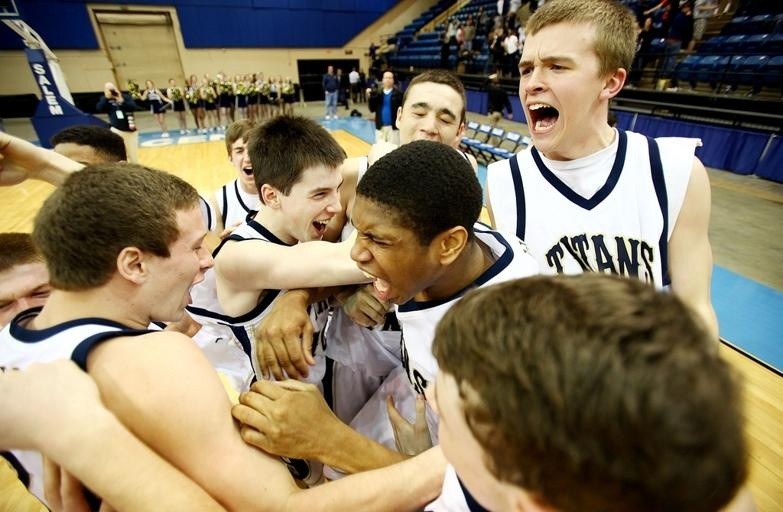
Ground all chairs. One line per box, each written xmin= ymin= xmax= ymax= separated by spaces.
xmin=472 ymin=128 xmax=505 ymax=168
xmin=458 ymin=121 xmax=479 ymax=162
xmin=502 ymin=136 xmax=532 ymax=160
xmin=462 ymin=125 xmax=492 ymax=163
xmin=486 ymin=132 xmax=521 ymax=163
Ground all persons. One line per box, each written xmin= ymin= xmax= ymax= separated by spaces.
xmin=208 ymin=120 xmax=262 ymax=253
xmin=360 ymin=71 xmax=367 ymax=101
xmin=348 ymin=67 xmax=358 ymax=104
xmin=0 ymin=127 xmax=216 ymax=233
xmin=366 ymin=70 xmax=404 ymax=144
xmin=137 ymin=72 xmax=296 ymax=137
xmin=97 ymin=82 xmax=139 ymax=164
xmin=232 ymin=141 xmax=542 ymax=512
xmin=0 ymin=361 xmax=225 ymax=512
xmin=441 ymin=2 xmax=719 ymax=80
xmin=321 ymin=65 xmax=338 ymax=120
xmin=185 ymin=116 xmax=399 ymax=488
xmin=1 ymin=163 xmax=449 ymax=512
xmin=219 ymin=71 xmax=478 ymax=423
xmin=485 ymin=0 xmax=718 ymax=350
xmin=0 ymin=233 xmax=116 ymax=512
xmin=425 ymin=276 xmax=747 ymax=511
xmin=336 ymin=71 xmax=348 ymax=106
xmin=486 ymin=75 xmax=513 ymax=119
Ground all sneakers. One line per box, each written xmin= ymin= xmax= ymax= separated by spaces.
xmin=187 ymin=130 xmax=190 ymax=134
xmin=202 ymin=128 xmax=207 ymax=134
xmin=333 ymin=115 xmax=337 ymax=118
xmin=161 ymin=133 xmax=165 ymax=137
xmin=165 ymin=132 xmax=168 ymax=137
xmin=325 ymin=115 xmax=329 ymax=119
xmin=197 ymin=129 xmax=201 ymax=134
xmin=216 ymin=126 xmax=220 ymax=131
xmin=180 ymin=129 xmax=184 ymax=134
xmin=209 ymin=127 xmax=214 ymax=133
